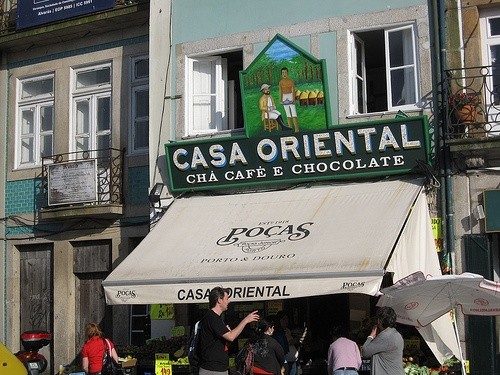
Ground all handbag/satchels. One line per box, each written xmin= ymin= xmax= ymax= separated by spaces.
xmin=100 ymin=338 xmax=124 ymax=375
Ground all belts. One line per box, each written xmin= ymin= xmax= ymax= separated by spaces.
xmin=337 ymin=367 xmax=359 ymax=374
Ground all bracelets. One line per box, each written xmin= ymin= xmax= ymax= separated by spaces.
xmin=368 ymin=336 xmax=373 ymax=339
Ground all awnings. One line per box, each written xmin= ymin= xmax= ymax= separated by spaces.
xmin=101 ymin=178 xmax=458 ymax=364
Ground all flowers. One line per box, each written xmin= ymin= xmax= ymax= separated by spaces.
xmin=449 ymin=92 xmax=482 ymax=109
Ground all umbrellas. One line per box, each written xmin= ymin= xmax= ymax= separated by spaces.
xmin=376 ymin=270 xmax=500 ymax=327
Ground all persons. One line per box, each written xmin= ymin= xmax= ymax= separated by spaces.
xmin=251 ymin=310 xmax=304 ymax=375
xmin=81 ymin=322 xmax=118 ymax=375
xmin=326 ymin=326 xmax=362 ymax=375
xmin=362 ymin=307 xmax=405 ymax=375
xmin=195 ymin=286 xmax=260 ymax=375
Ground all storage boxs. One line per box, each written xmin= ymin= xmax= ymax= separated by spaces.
xmin=118 ymin=358 xmax=136 ymax=368
xmin=20 ymin=331 xmax=52 ymax=349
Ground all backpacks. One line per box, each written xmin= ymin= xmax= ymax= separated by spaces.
xmin=362 ymin=359 xmax=372 ymax=371
xmin=188 ymin=316 xmax=226 ymax=367
xmin=234 ymin=335 xmax=257 ymax=375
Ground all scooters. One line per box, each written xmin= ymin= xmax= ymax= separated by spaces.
xmin=14 ymin=330 xmax=53 ymax=375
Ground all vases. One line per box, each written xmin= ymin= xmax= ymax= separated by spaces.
xmin=454 ymin=105 xmax=478 ymax=123
